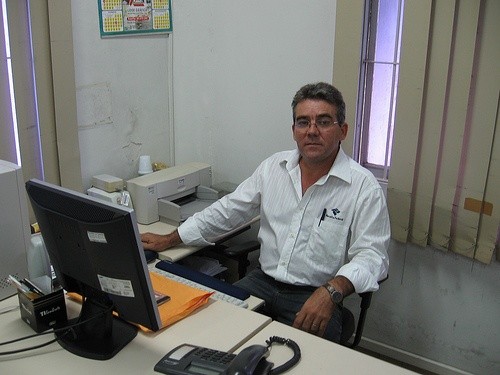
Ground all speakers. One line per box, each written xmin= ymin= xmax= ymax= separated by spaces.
xmin=87 ymin=187 xmax=134 ymax=209
xmin=26 ymin=236 xmax=52 ymax=280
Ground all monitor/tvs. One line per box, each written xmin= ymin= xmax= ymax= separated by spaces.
xmin=24 ymin=178 xmax=162 ymax=360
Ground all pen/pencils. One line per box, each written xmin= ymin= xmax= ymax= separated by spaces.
xmin=317 ymin=208 xmax=327 ymax=227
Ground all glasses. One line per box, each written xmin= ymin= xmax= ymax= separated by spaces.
xmin=295 ymin=119 xmax=340 ymax=129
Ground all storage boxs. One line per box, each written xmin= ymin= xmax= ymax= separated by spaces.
xmin=16 ymin=274 xmax=68 ymax=333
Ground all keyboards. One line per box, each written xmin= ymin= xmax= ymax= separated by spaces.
xmin=153 ymin=267 xmax=248 ymax=309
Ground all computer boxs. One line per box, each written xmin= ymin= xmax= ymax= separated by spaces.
xmin=0 ymin=160 xmax=30 ymax=302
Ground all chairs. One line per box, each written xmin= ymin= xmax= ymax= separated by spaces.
xmin=225 ymin=240 xmax=391 ymax=350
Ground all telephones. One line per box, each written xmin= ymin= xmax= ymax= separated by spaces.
xmin=153 ymin=337 xmax=274 ymax=375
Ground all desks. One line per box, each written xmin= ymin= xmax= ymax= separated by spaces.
xmin=232 ymin=319 xmax=420 ymax=375
xmin=0 ymin=271 xmax=272 ymax=375
xmin=136 ymin=215 xmax=260 ymax=269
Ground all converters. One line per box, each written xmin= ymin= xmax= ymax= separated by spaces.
xmin=93 ymin=174 xmax=122 ymax=192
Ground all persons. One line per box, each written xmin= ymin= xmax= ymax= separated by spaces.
xmin=139 ymin=82 xmax=391 ymax=344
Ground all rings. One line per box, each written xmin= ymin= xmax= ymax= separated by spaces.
xmin=312 ymin=323 xmax=319 ymax=329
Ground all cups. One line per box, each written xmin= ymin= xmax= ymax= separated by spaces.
xmin=138 ymin=156 xmax=153 ymax=174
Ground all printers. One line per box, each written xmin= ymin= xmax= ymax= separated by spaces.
xmin=127 ymin=162 xmax=220 ymax=225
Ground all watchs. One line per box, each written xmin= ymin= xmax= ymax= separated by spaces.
xmin=322 ymin=283 xmax=343 ymax=304
